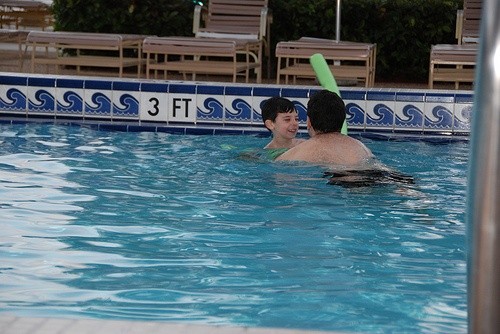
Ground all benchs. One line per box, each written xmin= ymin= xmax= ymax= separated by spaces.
xmin=429 ymin=44 xmax=479 ymax=90
xmin=0 ymin=30 xmax=376 ymax=88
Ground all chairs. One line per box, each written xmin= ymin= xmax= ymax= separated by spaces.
xmin=453 ymin=1 xmax=482 ymax=90
xmin=192 ymin=0 xmax=272 ymax=82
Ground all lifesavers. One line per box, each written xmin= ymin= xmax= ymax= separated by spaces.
xmin=310 ymin=53 xmax=347 ymax=136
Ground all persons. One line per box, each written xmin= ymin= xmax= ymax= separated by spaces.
xmin=261 ymin=95 xmax=306 ymax=149
xmin=275 ymin=89 xmax=372 ymax=165
